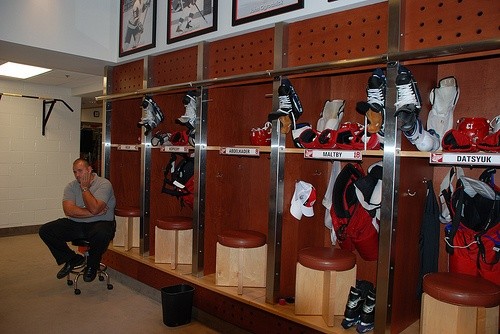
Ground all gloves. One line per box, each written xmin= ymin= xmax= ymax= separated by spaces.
xmin=291 ymin=121 xmax=384 ymax=151
xmin=438 ymin=117 xmax=500 ymax=153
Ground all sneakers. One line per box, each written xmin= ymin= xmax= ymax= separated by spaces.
xmin=137 ymin=97 xmax=164 ymax=136
xmin=355 ymin=68 xmax=387 ymax=137
xmin=268 ymin=78 xmax=303 ymax=134
xmin=426 ymin=76 xmax=460 ymax=151
xmin=175 ymin=90 xmax=198 ymax=132
xmin=316 ymin=98 xmax=345 ymax=134
xmin=394 ymin=65 xmax=422 ymax=132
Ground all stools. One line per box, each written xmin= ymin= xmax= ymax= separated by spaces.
xmin=295 ymin=247 xmax=357 ymax=326
xmin=114 ymin=207 xmax=141 ymax=251
xmin=155 ymin=216 xmax=193 ymax=269
xmin=66 ymin=239 xmax=114 ymax=295
xmin=216 ymin=229 xmax=267 ymax=294
xmin=419 ymin=272 xmax=500 ymax=334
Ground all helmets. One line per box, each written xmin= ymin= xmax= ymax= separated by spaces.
xmin=452 ymin=178 xmax=500 ymax=232
xmin=249 ymin=126 xmax=271 ymax=146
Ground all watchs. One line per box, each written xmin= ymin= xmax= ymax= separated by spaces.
xmin=82 ymin=188 xmax=89 ymax=192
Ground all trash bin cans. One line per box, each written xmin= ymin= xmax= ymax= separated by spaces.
xmin=161 ymin=284 xmax=195 ymax=327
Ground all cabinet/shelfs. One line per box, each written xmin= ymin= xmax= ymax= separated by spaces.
xmin=94 ymin=0 xmax=500 ymax=334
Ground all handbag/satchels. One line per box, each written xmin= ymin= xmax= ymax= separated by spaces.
xmin=161 ymin=151 xmax=195 ymax=196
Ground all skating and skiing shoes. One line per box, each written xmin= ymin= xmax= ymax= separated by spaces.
xmin=355 ymin=288 xmax=376 ymax=333
xmin=341 ymin=280 xmax=374 ymax=330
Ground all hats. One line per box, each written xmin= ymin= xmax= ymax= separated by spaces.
xmin=289 ymin=180 xmax=303 ymax=220
xmin=294 ymin=181 xmax=317 ymax=217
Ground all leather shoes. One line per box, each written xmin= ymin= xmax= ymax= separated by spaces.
xmin=56 ymin=254 xmax=84 ymax=279
xmin=83 ymin=255 xmax=102 ymax=282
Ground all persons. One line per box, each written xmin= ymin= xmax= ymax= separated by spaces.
xmin=176 ymin=0 xmax=197 ymax=32
xmin=39 ymin=158 xmax=116 ymax=281
xmin=124 ymin=0 xmax=150 ymax=50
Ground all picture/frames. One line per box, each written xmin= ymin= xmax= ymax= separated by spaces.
xmin=119 ymin=0 xmax=157 ymax=57
xmin=232 ymin=0 xmax=304 ymax=27
xmin=166 ymin=0 xmax=218 ymax=44
